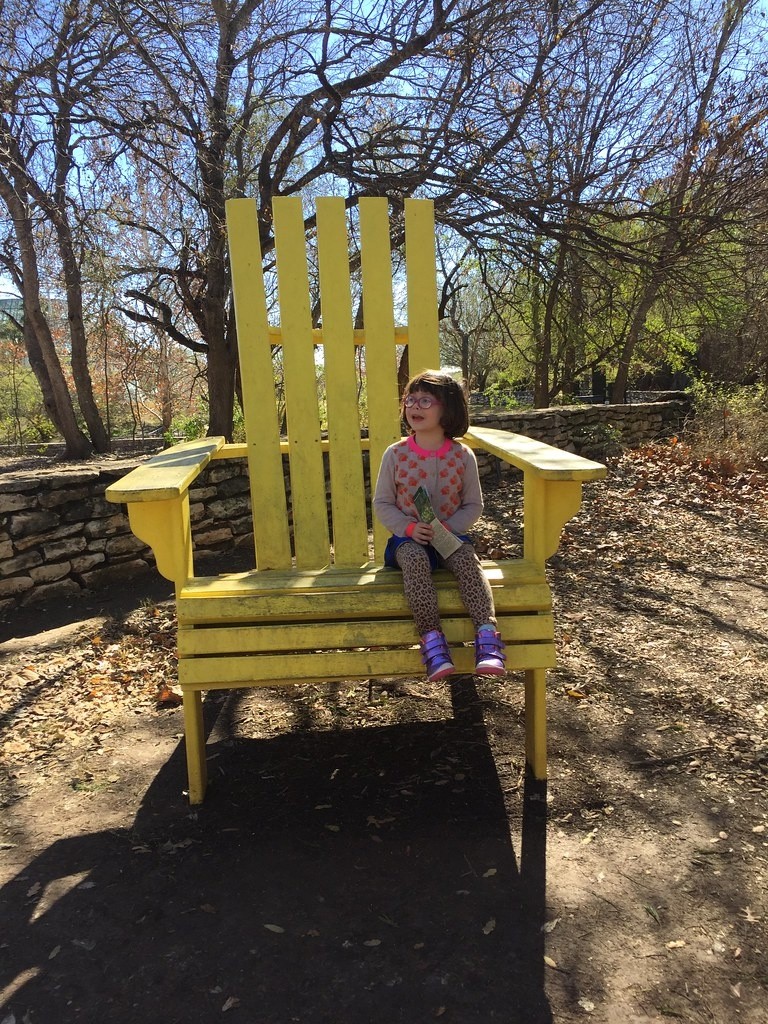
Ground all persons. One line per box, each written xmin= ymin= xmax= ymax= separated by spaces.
xmin=373 ymin=372 xmax=506 ymax=680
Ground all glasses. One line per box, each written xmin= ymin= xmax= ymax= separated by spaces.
xmin=403 ymin=395 xmax=443 ymax=409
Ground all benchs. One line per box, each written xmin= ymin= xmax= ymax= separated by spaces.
xmin=106 ymin=196 xmax=610 ymax=805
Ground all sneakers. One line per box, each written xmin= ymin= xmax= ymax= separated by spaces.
xmin=474 ymin=629 xmax=506 ymax=673
xmin=419 ymin=630 xmax=455 ymax=682
